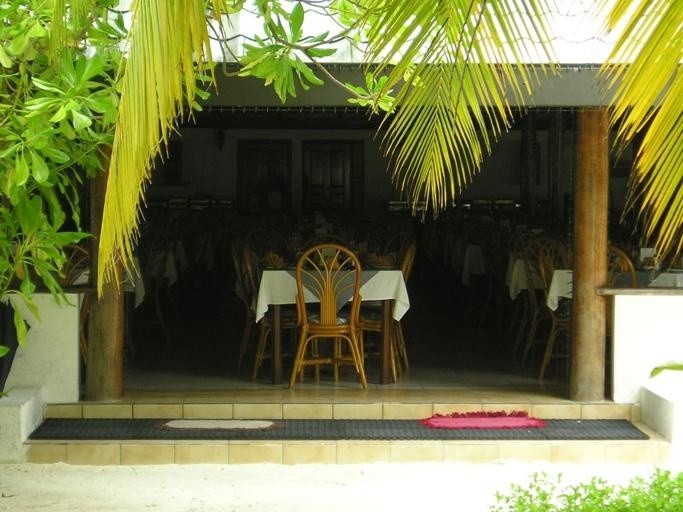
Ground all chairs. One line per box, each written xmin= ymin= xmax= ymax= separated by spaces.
xmin=233 ymin=226 xmax=417 ymax=390
xmin=512 ymin=227 xmax=636 ymax=383
xmin=56 ymin=230 xmax=170 ymax=370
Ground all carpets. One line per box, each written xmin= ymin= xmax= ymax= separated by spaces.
xmin=158 ymin=419 xmax=281 ymax=432
xmin=419 ymin=405 xmax=548 ymax=433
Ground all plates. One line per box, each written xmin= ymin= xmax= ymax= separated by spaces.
xmin=261 ymin=233 xmax=398 ymax=270
